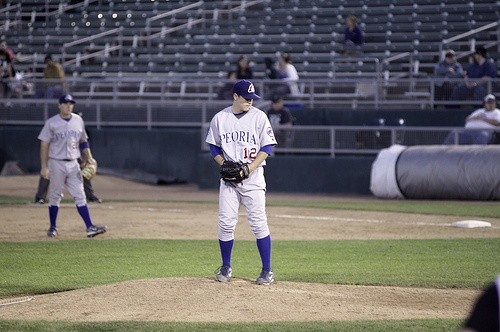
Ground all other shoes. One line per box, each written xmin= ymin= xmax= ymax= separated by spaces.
xmin=35 ymin=198 xmax=46 ymax=204
xmin=88 ymin=199 xmax=101 ymax=204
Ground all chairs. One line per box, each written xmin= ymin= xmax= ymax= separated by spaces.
xmin=0 ymin=0 xmax=500 ymax=99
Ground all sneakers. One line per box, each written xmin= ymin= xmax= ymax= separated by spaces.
xmin=256 ymin=271 xmax=275 ymax=285
xmin=47 ymin=226 xmax=57 ymax=237
xmin=87 ymin=225 xmax=108 ymax=238
xmin=214 ymin=265 xmax=232 ymax=282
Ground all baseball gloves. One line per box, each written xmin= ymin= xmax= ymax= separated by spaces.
xmin=81 ymin=159 xmax=97 ymax=181
xmin=220 ymin=161 xmax=250 ymax=183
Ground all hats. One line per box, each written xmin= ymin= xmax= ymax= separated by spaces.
xmin=59 ymin=93 xmax=76 ymax=104
xmin=232 ymin=80 xmax=261 ymax=99
xmin=445 ymin=50 xmax=458 ymax=56
xmin=484 ymin=94 xmax=496 ymax=102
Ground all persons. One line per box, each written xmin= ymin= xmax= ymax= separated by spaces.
xmin=216 ymin=71 xmax=241 ymax=102
xmin=36 ymin=94 xmax=108 ymax=237
xmin=206 ymin=79 xmax=278 ymax=286
xmin=431 ymin=43 xmax=500 ymax=145
xmin=33 ymin=54 xmax=67 ymax=98
xmin=233 ymin=55 xmax=254 ymax=80
xmin=0 ymin=36 xmax=27 ymax=97
xmin=335 ymin=15 xmax=364 ymax=63
xmin=266 ymin=95 xmax=293 ymax=125
xmin=264 ymin=51 xmax=299 ymax=100
xmin=35 ymin=111 xmax=102 ymax=203
